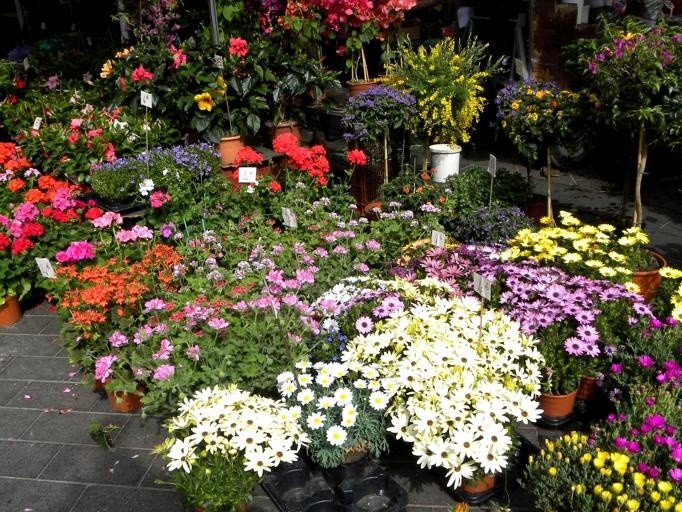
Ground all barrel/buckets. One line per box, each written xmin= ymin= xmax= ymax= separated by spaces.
xmin=428 ymin=143 xmax=462 ymax=183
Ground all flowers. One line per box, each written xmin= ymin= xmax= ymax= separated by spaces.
xmin=1 ymin=2 xmax=682 ymax=512
